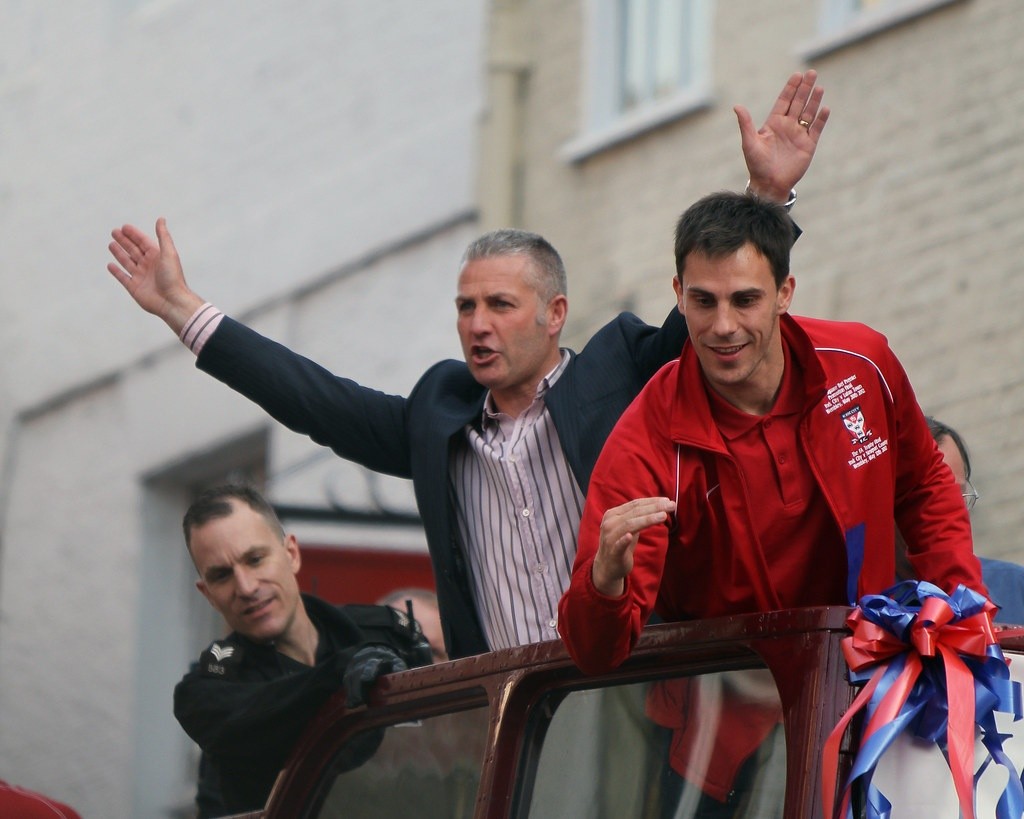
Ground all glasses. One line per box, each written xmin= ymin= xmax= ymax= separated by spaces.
xmin=957 ymin=478 xmax=978 ymax=512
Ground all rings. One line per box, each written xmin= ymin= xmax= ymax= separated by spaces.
xmin=799 ymin=120 xmax=809 ymax=126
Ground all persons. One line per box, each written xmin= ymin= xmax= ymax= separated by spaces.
xmin=174 ymin=486 xmax=445 ymax=819
xmin=559 ymin=193 xmax=1001 ymax=817
xmin=107 ymin=64 xmax=831 ymax=656
xmin=926 ymin=418 xmax=1024 ymax=624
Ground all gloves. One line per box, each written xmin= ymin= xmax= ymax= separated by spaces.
xmin=343 ymin=647 xmax=409 ymax=707
